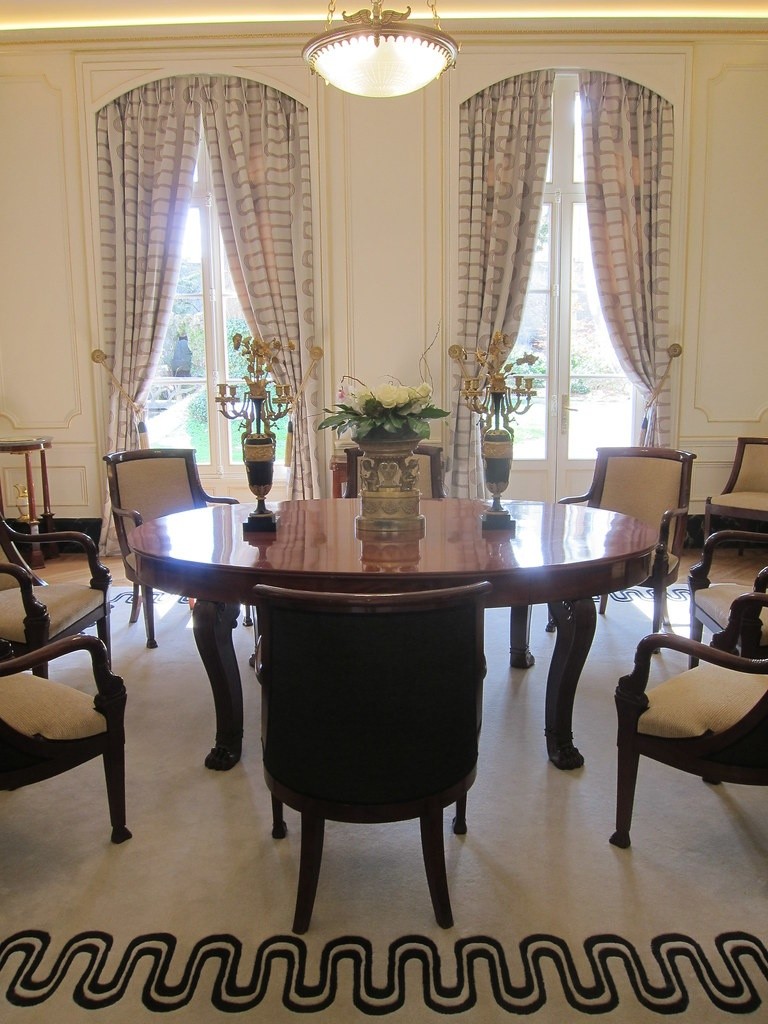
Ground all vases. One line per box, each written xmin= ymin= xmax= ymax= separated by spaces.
xmin=352 ymin=438 xmax=428 ymax=532
xmin=361 ymin=541 xmax=421 ymax=573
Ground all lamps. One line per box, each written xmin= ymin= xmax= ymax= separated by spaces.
xmin=301 ymin=0 xmax=462 ymax=98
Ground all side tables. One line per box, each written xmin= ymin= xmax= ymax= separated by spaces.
xmin=0 ymin=435 xmax=59 ymax=570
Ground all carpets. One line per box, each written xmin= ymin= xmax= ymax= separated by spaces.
xmin=0 ymin=584 xmax=768 ymax=1024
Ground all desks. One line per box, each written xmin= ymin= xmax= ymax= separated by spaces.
xmin=125 ymin=498 xmax=660 ymax=772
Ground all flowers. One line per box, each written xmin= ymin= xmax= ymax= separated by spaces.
xmin=318 ymin=319 xmax=452 ymax=443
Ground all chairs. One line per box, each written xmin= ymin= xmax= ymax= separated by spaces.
xmin=609 ymin=592 xmax=768 ymax=848
xmin=688 ymin=530 xmax=768 ymax=670
xmin=704 ymin=438 xmax=768 ymax=556
xmin=0 ymin=634 xmax=131 ymax=845
xmin=256 ymin=580 xmax=490 ymax=935
xmin=343 ymin=445 xmax=444 ymax=498
xmin=103 ymin=450 xmax=240 ymax=648
xmin=0 ymin=514 xmax=112 ymax=680
xmin=546 ymin=446 xmax=697 ymax=653
